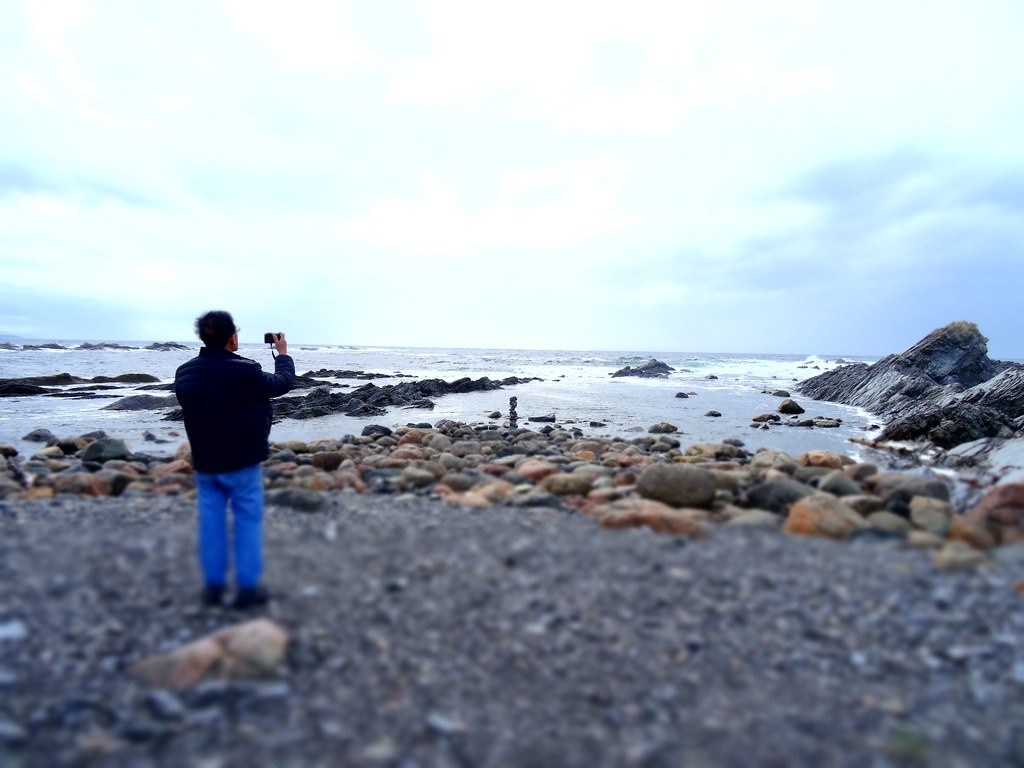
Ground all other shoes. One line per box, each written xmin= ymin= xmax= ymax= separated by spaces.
xmin=202 ymin=584 xmax=237 ymax=609
xmin=231 ymin=586 xmax=286 ymax=613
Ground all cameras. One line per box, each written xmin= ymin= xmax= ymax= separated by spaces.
xmin=264 ymin=333 xmax=280 ymax=343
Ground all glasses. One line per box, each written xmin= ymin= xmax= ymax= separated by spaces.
xmin=232 ymin=325 xmax=242 ymax=337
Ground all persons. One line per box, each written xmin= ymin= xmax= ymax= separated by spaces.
xmin=174 ymin=311 xmax=295 ymax=609
